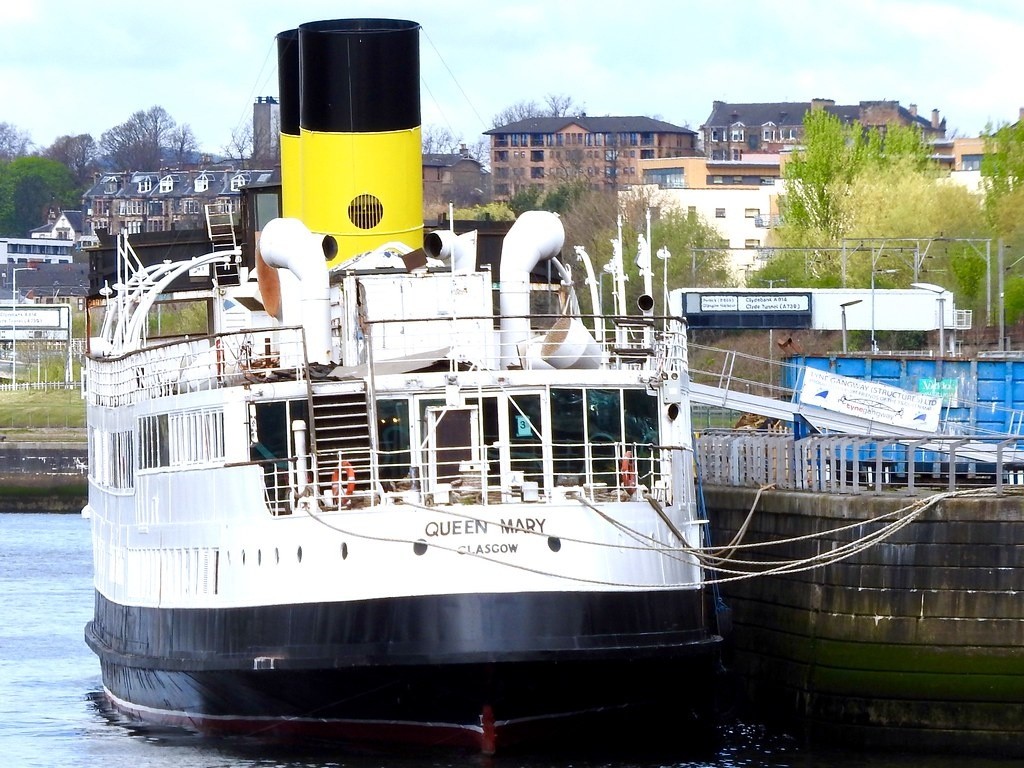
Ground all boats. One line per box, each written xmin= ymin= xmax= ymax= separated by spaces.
xmin=78 ymin=208 xmax=730 ymax=758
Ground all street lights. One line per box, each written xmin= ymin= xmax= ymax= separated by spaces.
xmin=11 ymin=267 xmax=39 ymax=393
xmin=839 ymin=299 xmax=862 ymax=353
xmin=871 ymin=269 xmax=902 ymax=353
xmin=912 ymin=283 xmax=946 ymax=358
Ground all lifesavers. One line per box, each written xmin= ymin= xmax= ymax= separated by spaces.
xmin=215 ymin=339 xmax=225 ymax=381
xmin=329 ymin=460 xmax=355 ymax=506
xmin=622 ymin=451 xmax=637 ymax=495
